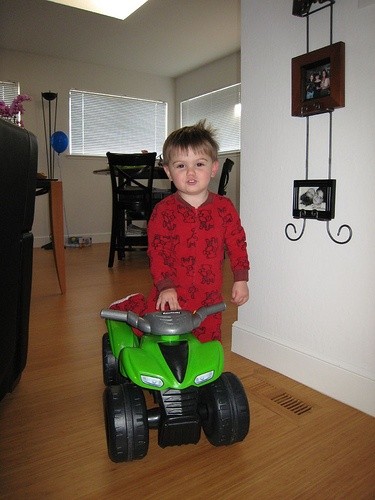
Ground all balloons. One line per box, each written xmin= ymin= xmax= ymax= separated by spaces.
xmin=51 ymin=131 xmax=69 ymax=154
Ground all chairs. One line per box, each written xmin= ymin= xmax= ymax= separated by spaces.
xmin=107 ymin=151 xmax=157 ymax=268
xmin=217 ymin=157 xmax=234 ymax=196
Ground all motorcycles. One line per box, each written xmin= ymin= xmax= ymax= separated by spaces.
xmin=102 ymin=302 xmax=250 ymax=463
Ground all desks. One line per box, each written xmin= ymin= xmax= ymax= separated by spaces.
xmin=36 ymin=178 xmax=67 ymax=295
xmin=93 ymin=168 xmax=177 ymax=227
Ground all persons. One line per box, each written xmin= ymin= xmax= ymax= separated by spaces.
xmin=306 ymin=69 xmax=330 ymax=90
xmin=301 ymin=188 xmax=326 ymax=211
xmin=107 ymin=120 xmax=248 ymax=345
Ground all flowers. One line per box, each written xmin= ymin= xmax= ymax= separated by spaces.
xmin=0 ymin=94 xmax=32 ymax=127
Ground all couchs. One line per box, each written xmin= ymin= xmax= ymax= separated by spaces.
xmin=0 ymin=118 xmax=38 ymax=401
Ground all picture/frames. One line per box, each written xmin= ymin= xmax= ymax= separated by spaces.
xmin=290 ymin=41 xmax=345 ymax=116
xmin=293 ymin=179 xmax=336 ymax=220
